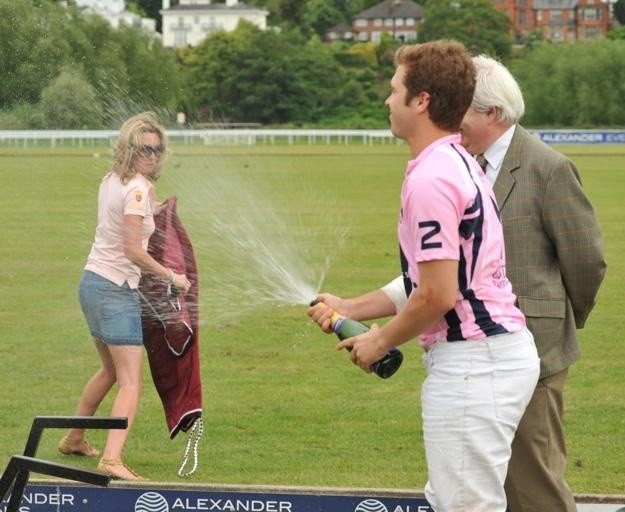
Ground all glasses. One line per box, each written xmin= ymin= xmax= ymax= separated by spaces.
xmin=130 ymin=144 xmax=164 ymax=159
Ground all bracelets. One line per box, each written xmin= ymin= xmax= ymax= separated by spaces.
xmin=163 ymin=270 xmax=175 ymax=284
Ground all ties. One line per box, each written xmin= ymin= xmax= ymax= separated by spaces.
xmin=476 ymin=153 xmax=489 ymax=173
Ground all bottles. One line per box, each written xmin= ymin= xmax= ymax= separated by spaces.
xmin=310 ymin=297 xmax=404 ymax=380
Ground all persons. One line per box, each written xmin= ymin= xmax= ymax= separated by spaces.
xmin=458 ymin=54 xmax=607 ymax=512
xmin=308 ymin=39 xmax=540 ymax=512
xmin=58 ymin=109 xmax=192 ymax=482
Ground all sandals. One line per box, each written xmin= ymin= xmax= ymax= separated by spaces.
xmin=94 ymin=457 xmax=151 ymax=485
xmin=56 ymin=437 xmax=102 ymax=458
